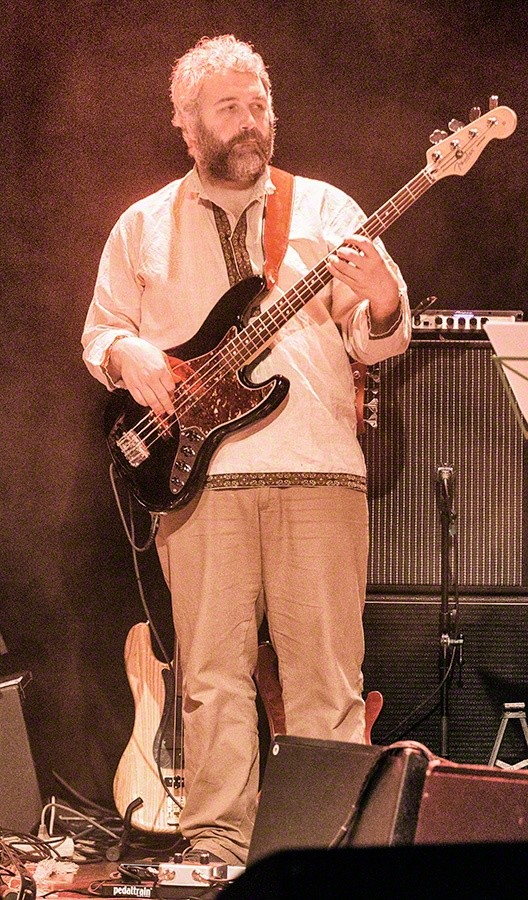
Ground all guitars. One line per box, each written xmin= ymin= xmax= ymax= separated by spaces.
xmin=256 ymin=639 xmax=382 ymax=797
xmin=105 ymin=96 xmax=518 ymax=516
xmin=112 ymin=557 xmax=271 ymax=829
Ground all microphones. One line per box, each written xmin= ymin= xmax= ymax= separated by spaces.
xmin=436 ymin=467 xmax=453 ymax=482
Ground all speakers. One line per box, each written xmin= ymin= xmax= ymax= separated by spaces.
xmin=0 ymin=669 xmax=50 ymax=839
xmin=355 ymin=341 xmax=528 ymax=597
xmin=358 ymin=591 xmax=528 ymax=769
xmin=240 ymin=734 xmax=528 ymax=870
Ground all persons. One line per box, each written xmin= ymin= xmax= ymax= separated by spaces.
xmin=81 ymin=33 xmax=412 ymax=866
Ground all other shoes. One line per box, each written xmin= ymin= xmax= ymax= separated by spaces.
xmin=183 ymin=848 xmax=222 ymax=862
